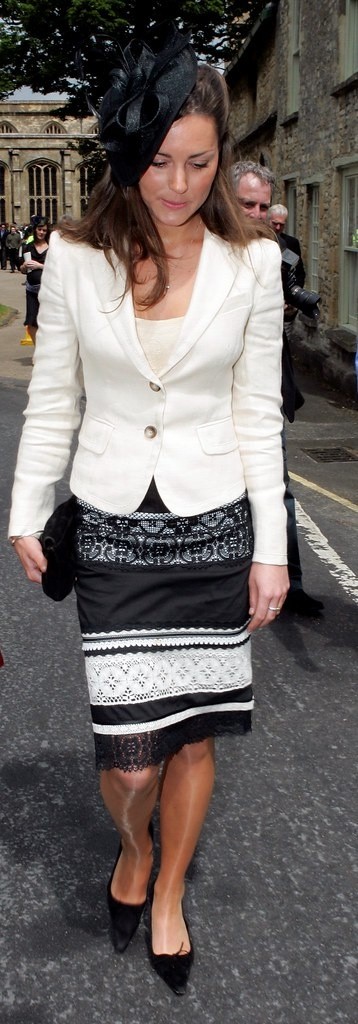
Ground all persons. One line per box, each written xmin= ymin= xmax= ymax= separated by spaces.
xmin=8 ymin=22 xmax=290 ymax=997
xmin=0 ymin=216 xmax=72 ymax=364
xmin=232 ymin=160 xmax=324 ymax=612
xmin=269 ymin=203 xmax=305 ymax=311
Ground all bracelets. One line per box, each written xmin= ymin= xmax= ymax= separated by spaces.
xmin=10 ymin=536 xmax=21 ymax=546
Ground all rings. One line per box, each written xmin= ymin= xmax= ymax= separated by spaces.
xmin=268 ymin=607 xmax=280 ymax=611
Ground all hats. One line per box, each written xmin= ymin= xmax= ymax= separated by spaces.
xmin=96 ymin=20 xmax=196 ymax=191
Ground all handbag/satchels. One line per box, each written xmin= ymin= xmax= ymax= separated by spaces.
xmin=37 ymin=496 xmax=81 ymax=603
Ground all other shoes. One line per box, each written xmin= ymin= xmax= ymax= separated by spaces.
xmin=148 ymin=876 xmax=192 ymax=995
xmin=279 ymin=587 xmax=324 ymax=614
xmin=108 ymin=822 xmax=157 ymax=953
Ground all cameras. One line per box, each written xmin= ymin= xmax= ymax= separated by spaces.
xmin=280 ymin=246 xmax=323 ymax=320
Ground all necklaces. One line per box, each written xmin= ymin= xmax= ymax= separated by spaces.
xmin=166 ymin=219 xmax=203 ymax=288
